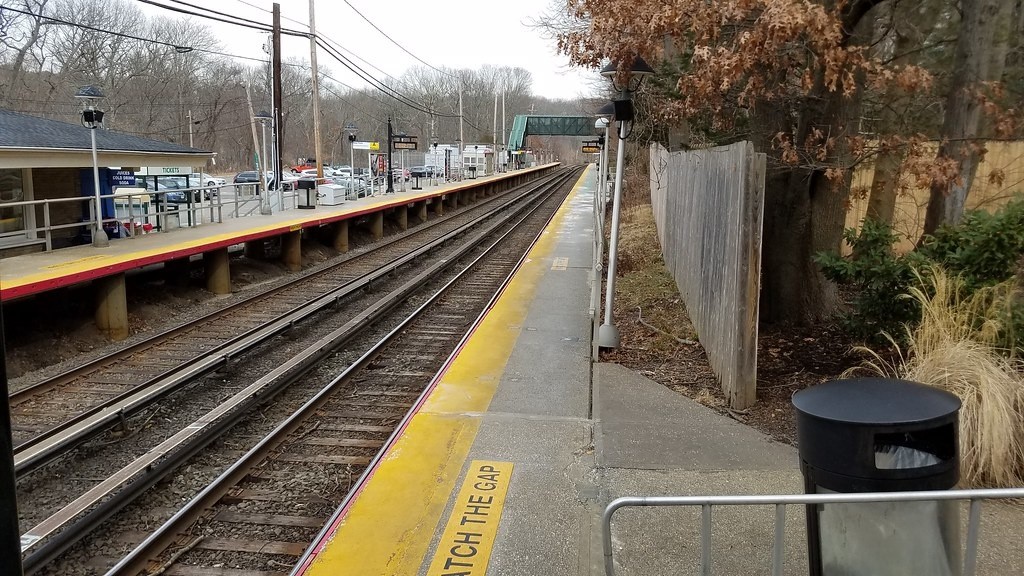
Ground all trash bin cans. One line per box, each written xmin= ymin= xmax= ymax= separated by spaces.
xmin=503 ymin=163 xmax=507 ymax=172
xmin=469 ymin=167 xmax=476 ymax=179
xmin=411 ymin=172 xmax=422 ymax=189
xmin=790 ymin=375 xmax=964 ymax=576
xmin=296 ymin=179 xmax=317 ymax=208
xmin=520 ymin=162 xmax=525 ymax=168
xmin=158 ymin=203 xmax=182 ymax=231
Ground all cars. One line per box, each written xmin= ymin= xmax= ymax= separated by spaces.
xmin=194 ymin=182 xmax=217 ymax=197
xmin=270 ymin=162 xmax=384 ymax=189
xmin=393 ymin=171 xmax=409 ymax=183
xmin=189 ymin=174 xmax=227 ymax=187
xmin=116 ymin=180 xmax=186 ymax=203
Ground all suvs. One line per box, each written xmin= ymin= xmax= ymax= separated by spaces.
xmin=158 ymin=179 xmax=208 ymax=203
xmin=411 ymin=166 xmax=440 ymax=179
xmin=232 ymin=171 xmax=290 ymax=191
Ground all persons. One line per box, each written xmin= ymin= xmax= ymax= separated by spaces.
xmin=117 ymin=221 xmax=130 ymax=237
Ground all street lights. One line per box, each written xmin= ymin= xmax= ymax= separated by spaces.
xmin=595 ymin=102 xmax=618 ymax=274
xmin=431 ymin=135 xmax=440 ymax=187
xmin=474 ymin=141 xmax=479 ymax=178
xmin=601 ymin=55 xmax=659 ymax=347
xmin=82 ymin=107 xmax=111 ymax=248
xmin=253 ymin=110 xmax=274 ymax=215
xmin=454 ymin=138 xmax=463 ymax=182
xmin=345 ymin=122 xmax=358 ymax=201
